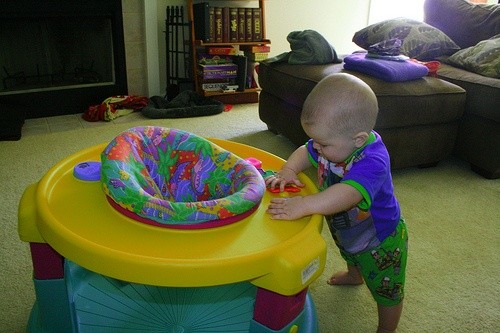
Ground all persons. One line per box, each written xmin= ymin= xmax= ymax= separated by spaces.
xmin=264 ymin=73 xmax=408 ymax=333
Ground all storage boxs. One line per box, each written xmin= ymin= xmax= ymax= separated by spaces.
xmin=197 ymin=45 xmax=270 ymax=90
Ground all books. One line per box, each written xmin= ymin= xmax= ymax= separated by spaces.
xmin=193 ymin=2 xmax=263 ymax=43
xmin=197 ymin=46 xmax=270 ymax=91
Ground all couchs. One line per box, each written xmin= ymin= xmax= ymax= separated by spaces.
xmin=258 ymin=55 xmax=500 ymax=180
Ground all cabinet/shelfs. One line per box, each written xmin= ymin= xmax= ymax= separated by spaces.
xmin=187 ymin=0 xmax=271 ymax=104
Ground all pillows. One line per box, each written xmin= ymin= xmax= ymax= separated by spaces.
xmin=352 ymin=0 xmax=500 ymax=79
